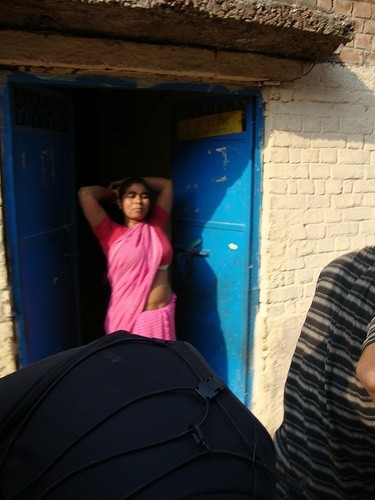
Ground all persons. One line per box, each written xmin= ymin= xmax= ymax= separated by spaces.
xmin=79 ymin=177 xmax=176 ymax=341
xmin=274 ymin=245 xmax=375 ymax=500
